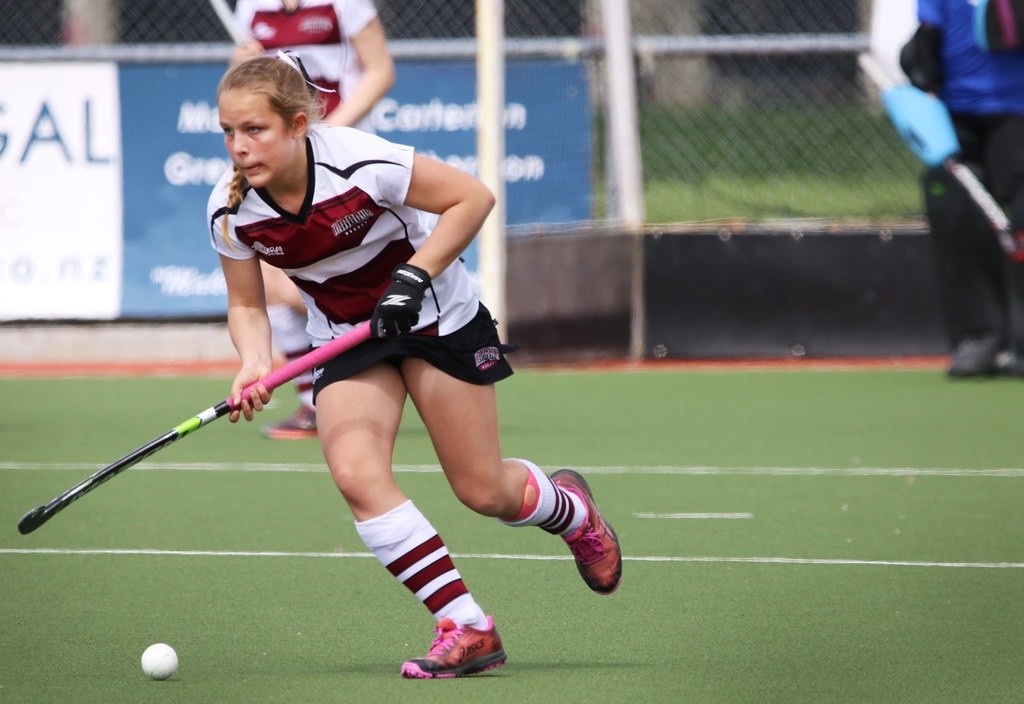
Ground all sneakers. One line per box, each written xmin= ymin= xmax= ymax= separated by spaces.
xmin=549 ymin=470 xmax=621 ymax=595
xmin=399 ymin=616 xmax=508 ymax=679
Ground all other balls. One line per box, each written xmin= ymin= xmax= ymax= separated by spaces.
xmin=141 ymin=643 xmax=178 ymax=681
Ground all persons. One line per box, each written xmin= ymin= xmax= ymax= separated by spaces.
xmin=232 ymin=0 xmax=398 ymax=441
xmin=206 ymin=48 xmax=620 ymax=681
xmin=868 ymin=0 xmax=1023 ymax=380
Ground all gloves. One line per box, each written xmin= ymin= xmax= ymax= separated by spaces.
xmin=369 ymin=265 xmax=431 ymax=342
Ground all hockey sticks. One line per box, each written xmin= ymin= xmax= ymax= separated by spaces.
xmin=18 ymin=321 xmax=372 ymax=537
xmin=861 ymin=52 xmax=1024 ymax=261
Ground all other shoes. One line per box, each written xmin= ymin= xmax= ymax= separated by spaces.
xmin=1002 ymin=351 xmax=1024 ymax=377
xmin=949 ymin=335 xmax=1001 ymax=376
